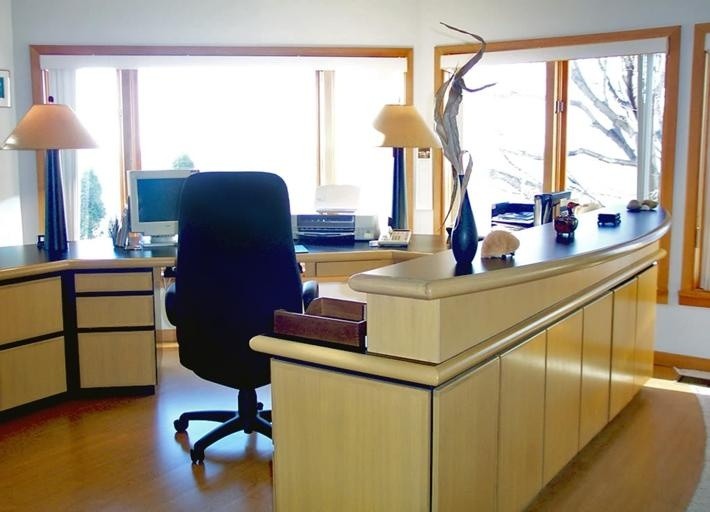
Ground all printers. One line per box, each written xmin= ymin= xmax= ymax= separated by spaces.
xmin=290 ymin=184 xmax=381 ymax=241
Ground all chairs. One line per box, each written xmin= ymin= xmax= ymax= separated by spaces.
xmin=162 ymin=168 xmax=321 ymax=466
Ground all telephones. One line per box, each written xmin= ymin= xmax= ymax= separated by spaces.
xmin=378 ymin=226 xmax=412 ymax=248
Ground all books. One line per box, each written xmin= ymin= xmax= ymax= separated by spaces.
xmin=533 ymin=191 xmax=578 ymax=225
xmin=491 ymin=211 xmax=534 ymax=233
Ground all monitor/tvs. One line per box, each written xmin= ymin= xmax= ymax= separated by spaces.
xmin=127 ymin=168 xmax=200 ymax=248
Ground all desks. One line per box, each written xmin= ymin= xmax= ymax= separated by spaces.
xmin=1 ymin=233 xmax=453 ymax=421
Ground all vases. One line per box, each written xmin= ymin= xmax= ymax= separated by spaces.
xmin=452 ymin=174 xmax=478 ymax=264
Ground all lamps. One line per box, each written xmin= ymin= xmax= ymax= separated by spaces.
xmin=0 ymin=94 xmax=99 ymax=263
xmin=372 ymin=100 xmax=444 ymax=236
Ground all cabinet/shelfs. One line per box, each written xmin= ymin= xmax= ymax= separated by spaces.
xmin=258 ymin=263 xmax=661 ymax=512
